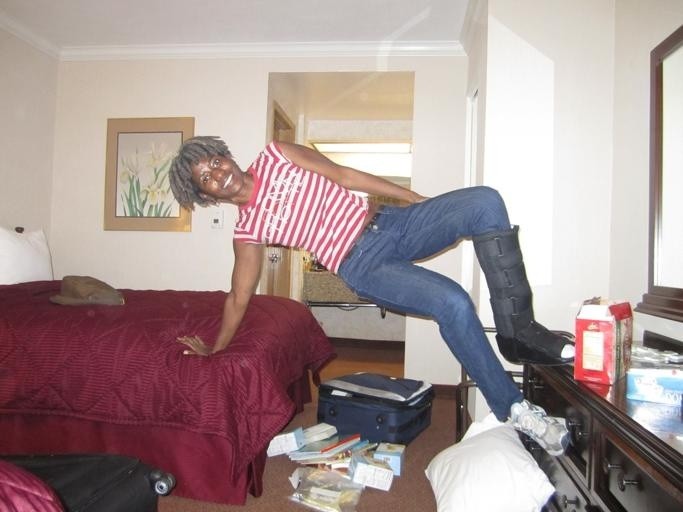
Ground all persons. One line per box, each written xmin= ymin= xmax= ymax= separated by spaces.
xmin=167 ymin=134 xmax=576 ymax=456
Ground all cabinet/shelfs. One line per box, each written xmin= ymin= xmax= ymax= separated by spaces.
xmin=516 ymin=330 xmax=682 ymax=511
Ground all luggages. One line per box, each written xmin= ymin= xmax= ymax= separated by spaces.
xmin=314 ymin=368 xmax=440 ymax=447
xmin=0 ymin=452 xmax=176 ymax=512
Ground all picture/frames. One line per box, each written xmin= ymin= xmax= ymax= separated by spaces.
xmin=102 ymin=116 xmax=194 ymax=233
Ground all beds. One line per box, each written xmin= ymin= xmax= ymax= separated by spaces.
xmin=0 ymin=279 xmax=305 ymax=505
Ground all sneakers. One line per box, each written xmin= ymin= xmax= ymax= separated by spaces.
xmin=505 ymin=395 xmax=571 ymax=456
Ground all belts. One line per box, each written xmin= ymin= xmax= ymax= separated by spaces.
xmin=342 ymin=204 xmax=385 ymax=264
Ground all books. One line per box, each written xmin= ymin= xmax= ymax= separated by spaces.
xmin=265 ymin=420 xmax=395 ymax=512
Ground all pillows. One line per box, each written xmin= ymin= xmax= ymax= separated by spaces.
xmin=423 ymin=425 xmax=555 ymax=511
xmin=0 ymin=227 xmax=54 ymax=286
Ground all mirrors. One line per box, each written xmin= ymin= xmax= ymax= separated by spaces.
xmin=633 ymin=24 xmax=682 ymax=324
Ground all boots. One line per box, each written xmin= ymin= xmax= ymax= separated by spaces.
xmin=469 ymin=220 xmax=581 ymax=367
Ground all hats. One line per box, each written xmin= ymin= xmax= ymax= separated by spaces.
xmin=49 ymin=274 xmax=125 ymax=307
